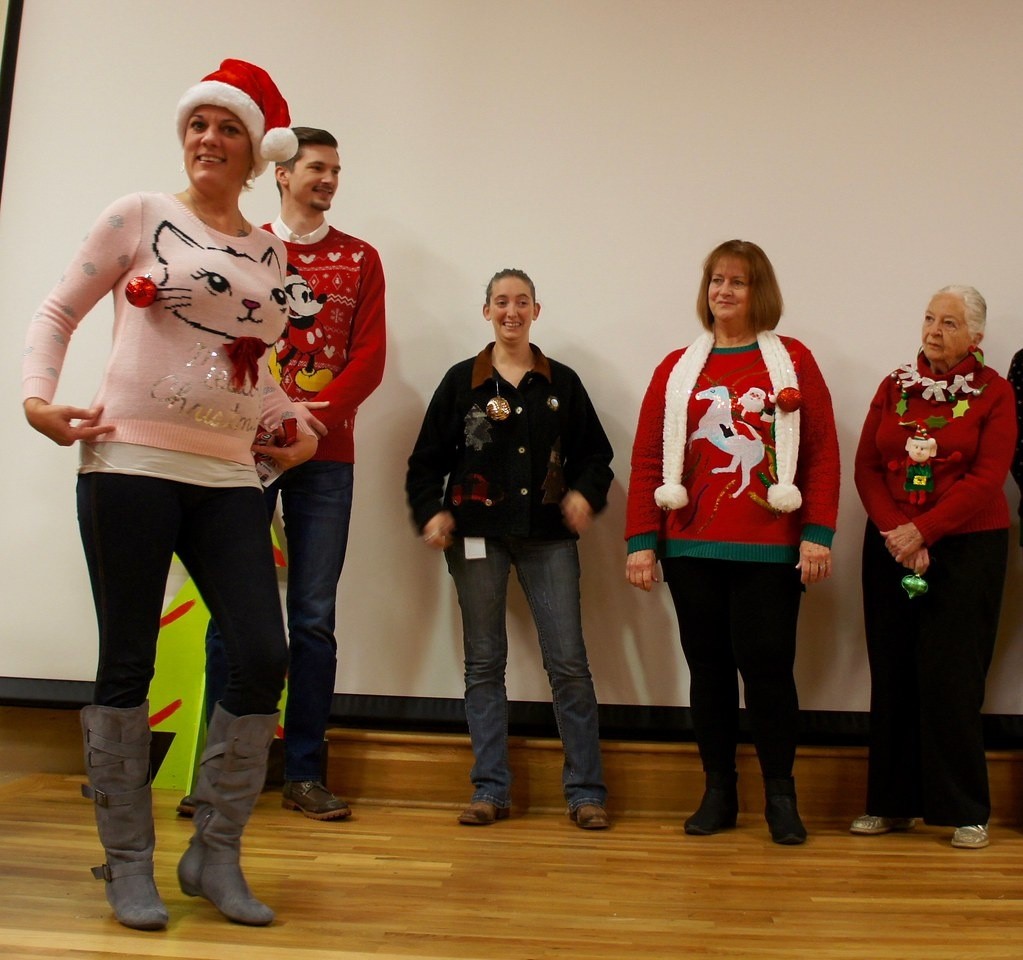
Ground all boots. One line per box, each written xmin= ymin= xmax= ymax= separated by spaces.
xmin=79 ymin=700 xmax=170 ymax=930
xmin=178 ymin=700 xmax=277 ymax=927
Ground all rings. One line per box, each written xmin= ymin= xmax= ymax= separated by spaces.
xmin=819 ymin=564 xmax=828 ymax=571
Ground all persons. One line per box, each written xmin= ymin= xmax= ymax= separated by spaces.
xmin=1006 ymin=344 xmax=1023 ymax=495
xmin=850 ymin=282 xmax=1019 ymax=852
xmin=17 ymin=57 xmax=321 ymax=934
xmin=617 ymin=235 xmax=845 ymax=849
xmin=177 ymin=123 xmax=358 ymax=824
xmin=401 ymin=266 xmax=619 ymax=831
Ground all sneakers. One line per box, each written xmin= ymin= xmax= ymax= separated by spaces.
xmin=569 ymin=804 xmax=609 ymax=829
xmin=683 ymin=771 xmax=739 ymax=835
xmin=764 ymin=777 xmax=808 ymax=843
xmin=176 ymin=794 xmax=197 ymax=815
xmin=282 ymin=778 xmax=352 ymax=822
xmin=458 ymin=801 xmax=510 ymax=824
xmin=952 ymin=825 xmax=990 ymax=849
xmin=849 ymin=814 xmax=917 ymax=834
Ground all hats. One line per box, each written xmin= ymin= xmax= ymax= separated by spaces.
xmin=176 ymin=58 xmax=299 ymax=182
